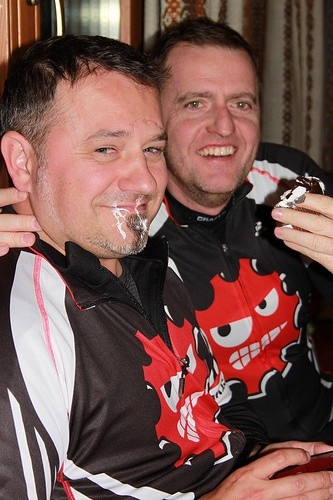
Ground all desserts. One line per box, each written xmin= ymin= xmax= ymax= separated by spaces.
xmin=245 ymin=160 xmax=327 ymax=233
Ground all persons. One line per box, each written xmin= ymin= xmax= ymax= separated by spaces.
xmin=0 ymin=34 xmax=332 ymax=500
xmin=1 ymin=17 xmax=332 ymax=459
